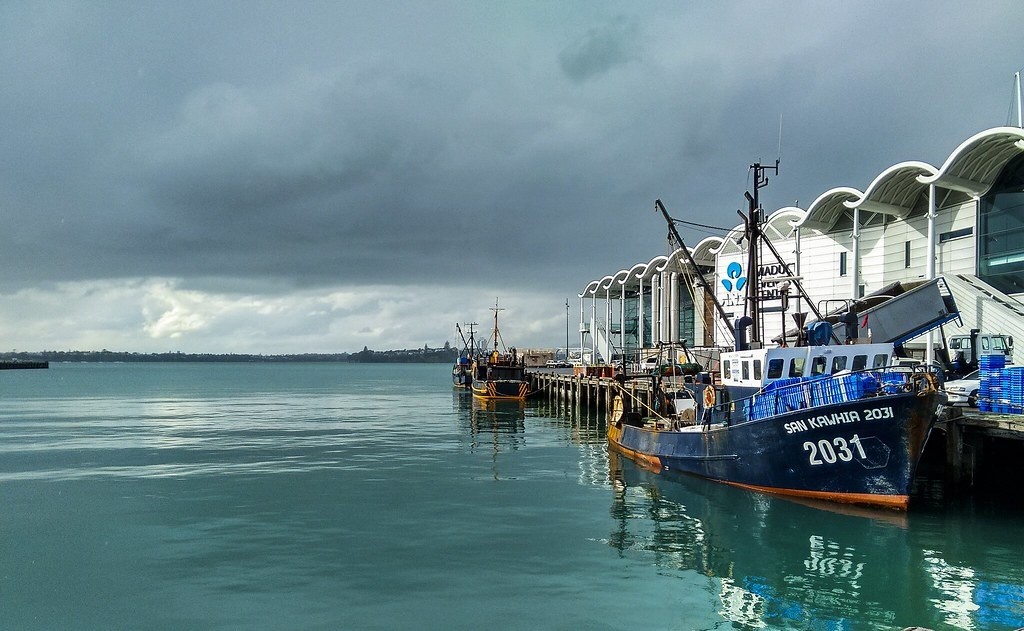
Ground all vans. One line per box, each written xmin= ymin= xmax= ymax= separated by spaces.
xmin=947 ymin=333 xmax=1015 ymax=365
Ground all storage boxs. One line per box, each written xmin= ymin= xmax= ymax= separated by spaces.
xmin=976 ymin=355 xmax=1024 ymax=414
xmin=744 ymin=373 xmax=928 ymax=421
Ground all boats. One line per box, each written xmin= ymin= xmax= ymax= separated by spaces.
xmin=454 ymin=321 xmax=483 ymax=388
xmin=605 ymin=122 xmax=951 ymax=511
xmin=471 ymin=297 xmax=533 ymax=399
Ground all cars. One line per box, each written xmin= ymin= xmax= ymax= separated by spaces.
xmin=939 ymin=364 xmax=1024 ymax=407
xmin=545 ymin=359 xmax=574 ymax=368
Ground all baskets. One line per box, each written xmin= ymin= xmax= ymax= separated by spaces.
xmin=977 ymin=355 xmax=1024 ymax=414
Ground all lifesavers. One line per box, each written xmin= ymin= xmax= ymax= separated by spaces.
xmin=704 ymin=385 xmax=716 ymax=407
